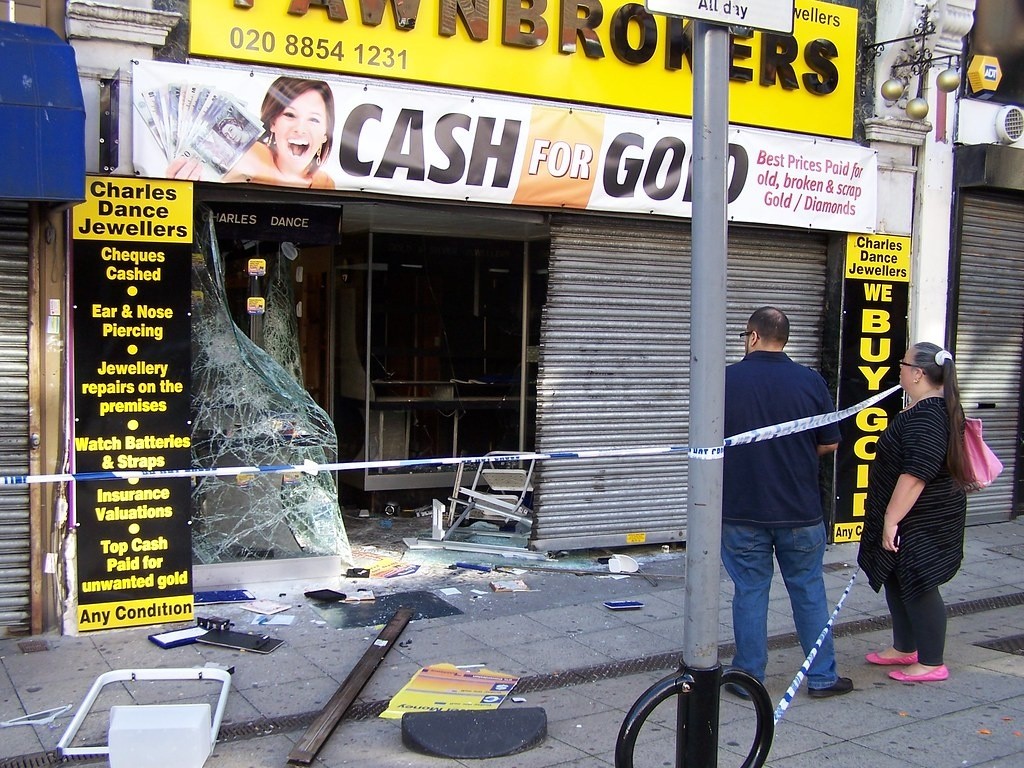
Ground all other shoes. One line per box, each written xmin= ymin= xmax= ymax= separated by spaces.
xmin=866 ymin=651 xmax=919 ymax=665
xmin=888 ymin=663 xmax=949 ymax=682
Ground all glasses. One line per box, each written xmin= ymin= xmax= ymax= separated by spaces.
xmin=739 ymin=331 xmax=760 ymax=340
xmin=899 ymin=359 xmax=926 ymax=376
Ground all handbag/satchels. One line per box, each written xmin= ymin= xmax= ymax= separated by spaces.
xmin=963 ymin=416 xmax=1003 ymax=492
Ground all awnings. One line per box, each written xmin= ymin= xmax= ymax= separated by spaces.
xmin=0 ymin=20 xmax=87 ymax=216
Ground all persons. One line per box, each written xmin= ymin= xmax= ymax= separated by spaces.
xmin=216 ymin=117 xmax=252 ymax=146
xmin=166 ymin=75 xmax=336 ymax=189
xmin=723 ymin=306 xmax=852 ymax=699
xmin=857 ymin=342 xmax=965 ymax=682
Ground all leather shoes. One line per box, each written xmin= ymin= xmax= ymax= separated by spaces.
xmin=724 ymin=684 xmax=752 ymax=700
xmin=808 ymin=676 xmax=853 ymax=698
